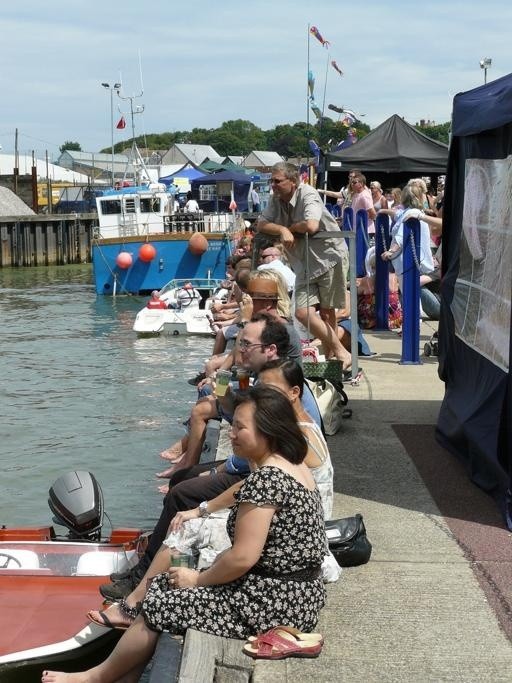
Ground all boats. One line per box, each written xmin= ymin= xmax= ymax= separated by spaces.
xmin=131 ymin=277 xmax=229 ymax=338
xmin=0 ymin=466 xmax=155 ymax=683
xmin=92 ymin=90 xmax=249 ymax=298
xmin=132 ymin=333 xmax=219 ymax=429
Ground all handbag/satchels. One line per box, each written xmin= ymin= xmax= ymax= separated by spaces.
xmin=325 ymin=513 xmax=372 ymax=568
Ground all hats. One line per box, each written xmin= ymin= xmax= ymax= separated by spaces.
xmin=242 ymin=278 xmax=283 ymax=302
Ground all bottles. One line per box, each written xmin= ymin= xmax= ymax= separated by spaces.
xmin=204 ymin=314 xmax=220 ymax=332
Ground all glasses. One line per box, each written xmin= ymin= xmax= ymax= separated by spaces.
xmin=237 ymin=340 xmax=268 ymax=350
xmin=269 ymin=176 xmax=290 ymax=184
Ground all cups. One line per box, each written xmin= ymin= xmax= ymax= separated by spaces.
xmin=237 ymin=370 xmax=251 ymax=388
xmin=211 ymin=370 xmax=235 ymax=398
xmin=169 ymin=547 xmax=199 ymax=570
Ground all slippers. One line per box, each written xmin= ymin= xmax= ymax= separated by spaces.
xmin=158 ymin=441 xmax=184 ymax=460
xmin=342 ymin=366 xmax=364 ymax=382
xmin=243 ymin=625 xmax=325 ymax=658
xmin=86 ymin=609 xmax=126 ymax=631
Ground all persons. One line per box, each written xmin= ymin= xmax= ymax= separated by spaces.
xmin=40 ymin=386 xmax=327 ymax=682
xmin=185 ymin=193 xmax=199 ymax=212
xmin=252 ymin=186 xmax=260 ymax=211
xmin=88 ymin=230 xmax=333 ymax=631
xmin=261 ymin=160 xmax=353 ymax=371
xmin=318 ymin=169 xmax=444 ymax=289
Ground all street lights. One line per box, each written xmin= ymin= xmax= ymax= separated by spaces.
xmin=479 ymin=56 xmax=493 ymax=86
xmin=102 ymin=82 xmax=122 ymax=190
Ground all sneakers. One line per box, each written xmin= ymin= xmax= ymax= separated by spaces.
xmin=189 ymin=371 xmax=205 ymax=385
xmin=110 ymin=571 xmax=132 ymax=582
xmin=99 ymin=582 xmax=130 ymax=603
